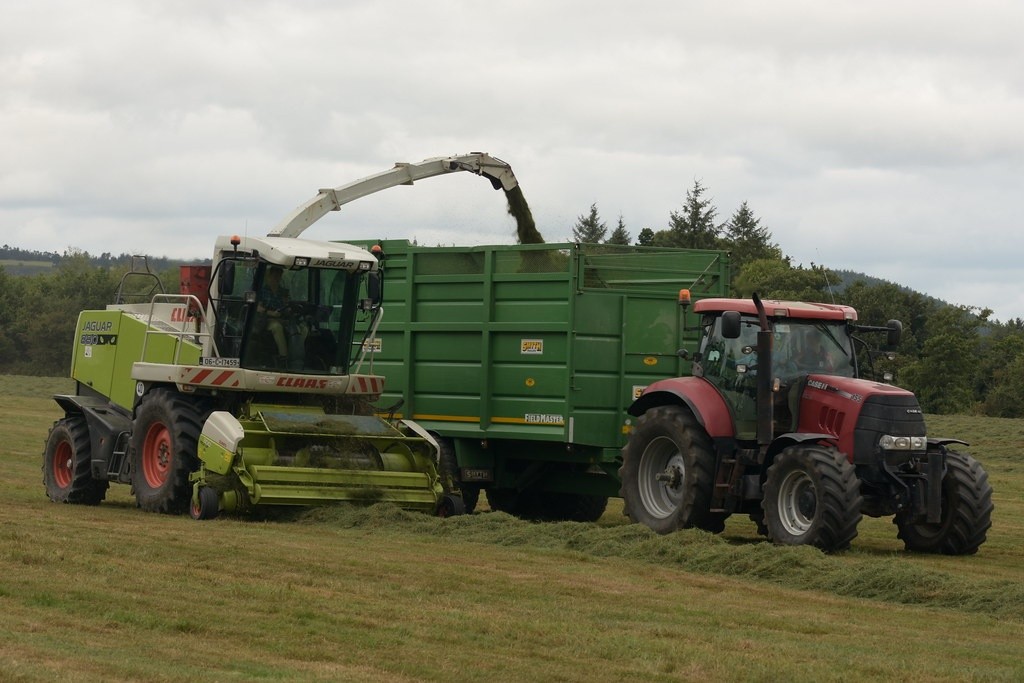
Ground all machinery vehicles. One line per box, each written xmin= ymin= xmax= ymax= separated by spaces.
xmin=39 ymin=146 xmax=548 ymax=521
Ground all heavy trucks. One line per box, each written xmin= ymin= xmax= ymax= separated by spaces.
xmin=321 ymin=236 xmax=994 ymax=555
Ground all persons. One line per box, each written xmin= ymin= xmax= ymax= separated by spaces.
xmin=747 ymin=329 xmax=835 ymax=380
xmin=256 ymin=266 xmax=309 ymax=372
